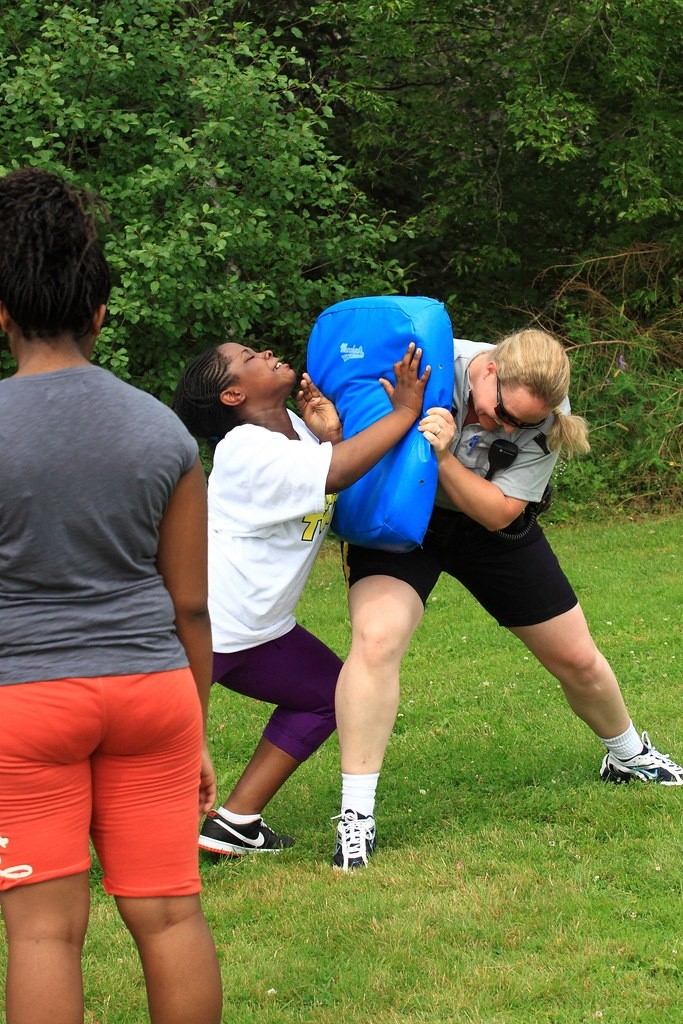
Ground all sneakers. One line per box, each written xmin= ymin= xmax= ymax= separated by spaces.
xmin=333 ymin=809 xmax=376 ymax=873
xmin=600 ymin=745 xmax=682 ymax=788
xmin=197 ymin=807 xmax=296 ymax=856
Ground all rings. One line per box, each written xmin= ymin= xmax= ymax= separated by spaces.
xmin=434 ymin=427 xmax=441 ymax=435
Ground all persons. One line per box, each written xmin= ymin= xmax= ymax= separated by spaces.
xmin=175 ymin=343 xmax=431 ymax=857
xmin=336 ymin=328 xmax=683 ymax=870
xmin=1 ymin=168 xmax=223 ymax=1024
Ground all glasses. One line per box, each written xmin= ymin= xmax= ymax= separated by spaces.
xmin=494 ymin=373 xmax=547 ymax=430
